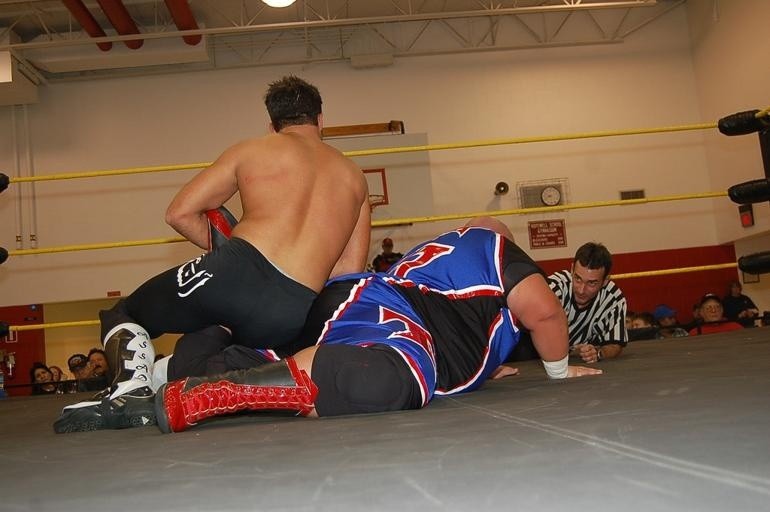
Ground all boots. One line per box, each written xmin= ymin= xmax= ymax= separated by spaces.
xmin=206 ymin=206 xmax=238 ymax=250
xmin=53 ymin=323 xmax=157 ymax=433
xmin=154 ymin=357 xmax=319 ymax=433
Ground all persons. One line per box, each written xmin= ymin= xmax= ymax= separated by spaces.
xmin=370 ymin=237 xmax=405 ymax=275
xmin=505 ymin=240 xmax=629 ymax=365
xmin=31 ymin=349 xmax=165 ymax=395
xmin=152 ymin=214 xmax=610 ymax=436
xmin=51 ymin=75 xmax=374 ymax=434
xmin=625 ymin=282 xmax=769 ymax=340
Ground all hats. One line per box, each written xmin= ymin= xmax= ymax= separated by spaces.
xmin=382 ymin=239 xmax=393 ymax=248
xmin=653 ymin=306 xmax=676 ymax=321
xmin=702 ymin=294 xmax=720 ymax=304
